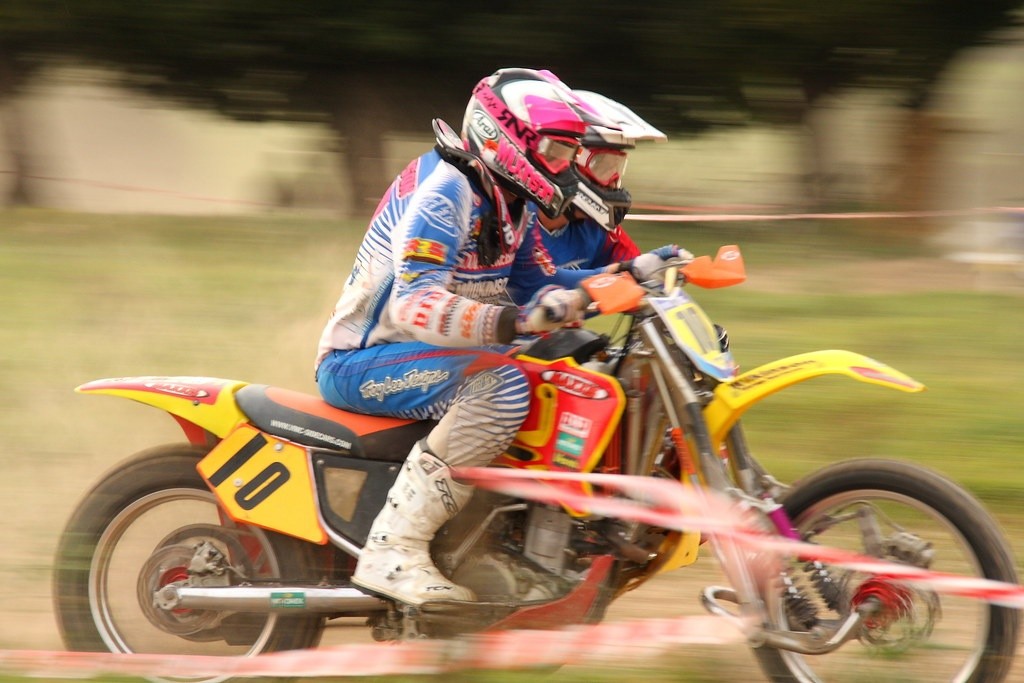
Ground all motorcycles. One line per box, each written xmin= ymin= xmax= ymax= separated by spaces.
xmin=49 ymin=242 xmax=1024 ymax=682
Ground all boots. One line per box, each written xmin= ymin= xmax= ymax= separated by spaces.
xmin=347 ymin=437 xmax=480 ymax=611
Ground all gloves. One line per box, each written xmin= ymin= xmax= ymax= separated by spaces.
xmin=628 ymin=242 xmax=695 ymax=296
xmin=515 ymin=284 xmax=585 ymax=338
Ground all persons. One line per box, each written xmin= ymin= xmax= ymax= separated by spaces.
xmin=315 ymin=67 xmax=697 ymax=613
xmin=505 ymin=91 xmax=669 ymax=312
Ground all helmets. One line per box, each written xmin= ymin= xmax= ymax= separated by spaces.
xmin=461 ymin=66 xmax=626 ymax=221
xmin=571 ymin=89 xmax=669 ymax=233
